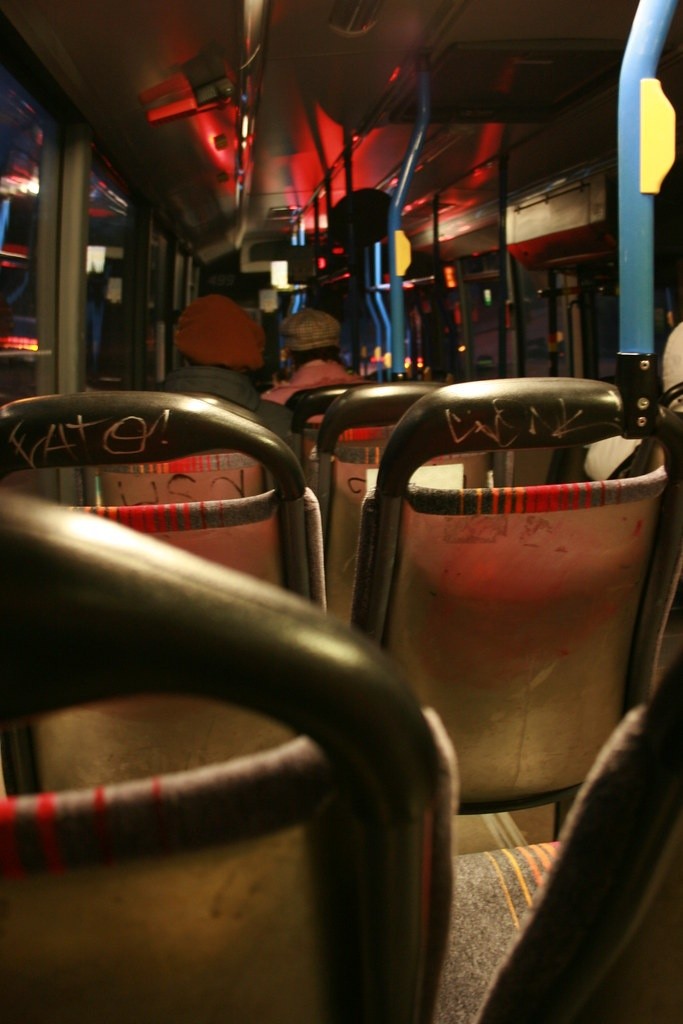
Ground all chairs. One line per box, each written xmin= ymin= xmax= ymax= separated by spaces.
xmin=0 ymin=378 xmax=683 ymax=1024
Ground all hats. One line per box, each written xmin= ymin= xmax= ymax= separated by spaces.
xmin=174 ymin=294 xmax=265 ymax=372
xmin=280 ymin=305 xmax=341 ymax=352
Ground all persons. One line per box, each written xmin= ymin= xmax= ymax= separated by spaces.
xmin=663 ymin=322 xmax=683 ymax=414
xmin=161 ymin=293 xmax=364 ymax=425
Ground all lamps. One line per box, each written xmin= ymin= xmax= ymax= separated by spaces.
xmin=179 ymin=49 xmax=241 ymax=108
